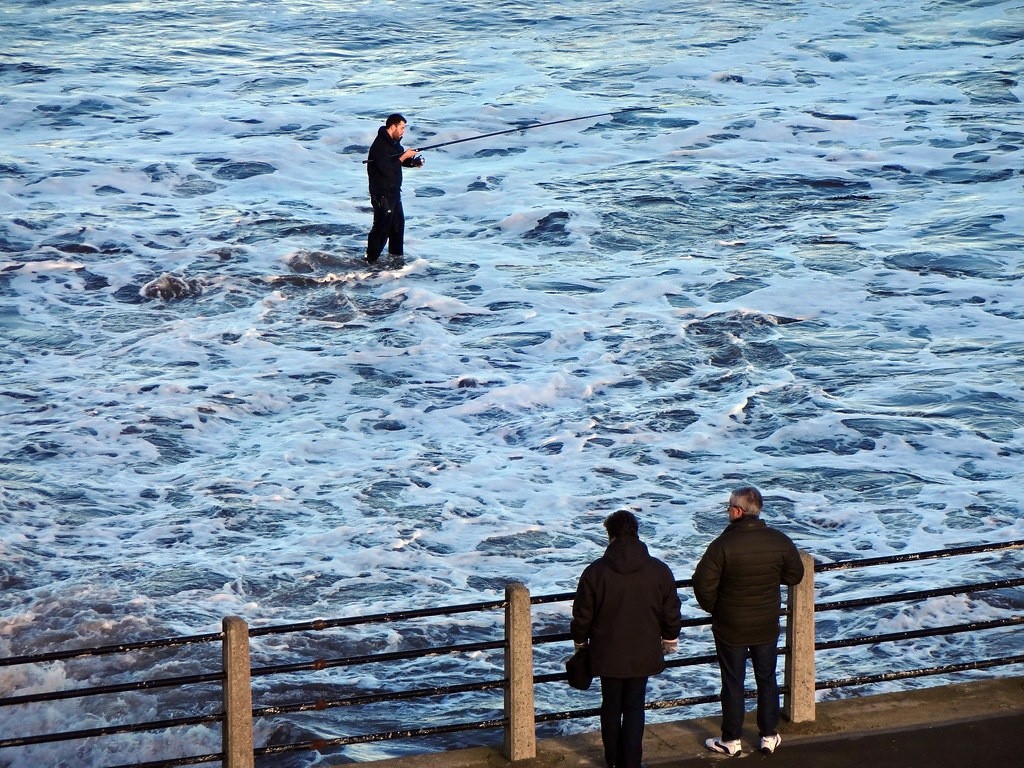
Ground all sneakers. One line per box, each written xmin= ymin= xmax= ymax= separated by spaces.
xmin=760 ymin=733 xmax=781 ymax=756
xmin=705 ymin=737 xmax=742 ymax=757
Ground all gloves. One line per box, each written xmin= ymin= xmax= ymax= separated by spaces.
xmin=574 ymin=640 xmax=588 ymax=654
xmin=661 ymin=638 xmax=678 ymax=655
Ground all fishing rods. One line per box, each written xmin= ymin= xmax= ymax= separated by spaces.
xmin=361 ymin=104 xmax=660 ymax=168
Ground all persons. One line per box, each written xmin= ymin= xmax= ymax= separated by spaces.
xmin=570 ymin=510 xmax=683 ymax=768
xmin=693 ymin=486 xmax=804 ymax=758
xmin=362 ymin=114 xmax=424 ymax=268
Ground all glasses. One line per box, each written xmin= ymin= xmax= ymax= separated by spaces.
xmin=726 ymin=503 xmax=746 ymax=512
